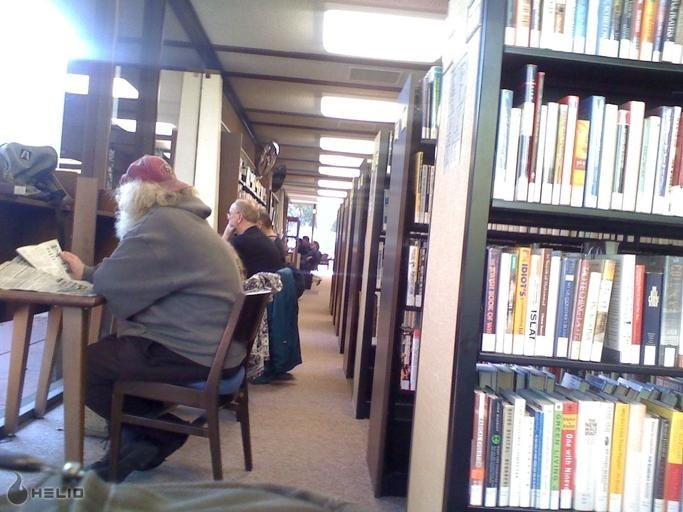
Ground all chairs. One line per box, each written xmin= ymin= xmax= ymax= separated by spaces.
xmin=110 ymin=291 xmax=272 ymax=480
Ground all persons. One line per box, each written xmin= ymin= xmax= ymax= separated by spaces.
xmin=58 ymin=154 xmax=247 ymax=476
xmin=257 ymin=214 xmax=286 ymax=254
xmin=221 ymin=198 xmax=281 ymax=342
xmin=297 ymin=234 xmax=323 ymax=273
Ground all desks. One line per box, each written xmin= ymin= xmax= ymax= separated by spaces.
xmin=0 ymin=287 xmax=106 ymax=463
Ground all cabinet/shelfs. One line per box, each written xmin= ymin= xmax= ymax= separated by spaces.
xmin=328 ymin=0 xmax=682 ymax=512
xmin=218 ymin=132 xmax=287 ymax=238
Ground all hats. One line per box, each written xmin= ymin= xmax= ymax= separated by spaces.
xmin=119 ymin=155 xmax=192 ymax=192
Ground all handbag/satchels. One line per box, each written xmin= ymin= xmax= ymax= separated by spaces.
xmin=0 ymin=447 xmax=367 ymax=512
xmin=0 ymin=142 xmax=72 ymax=200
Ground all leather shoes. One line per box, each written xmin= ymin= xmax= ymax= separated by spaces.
xmin=96 ymin=412 xmax=191 ymax=483
